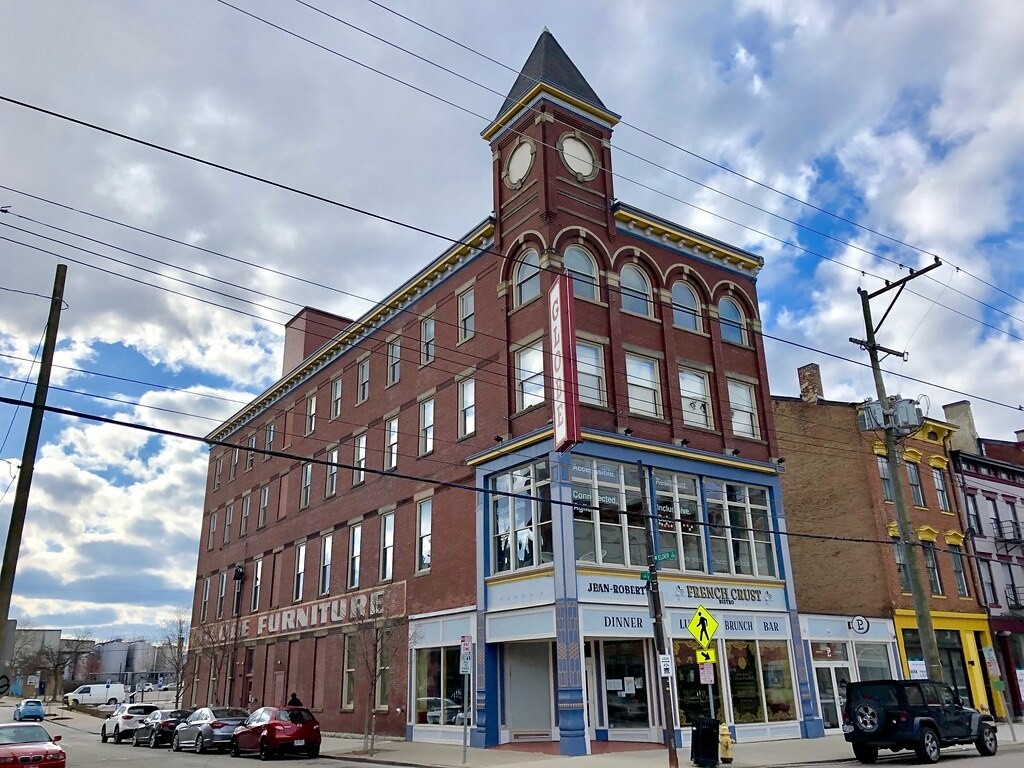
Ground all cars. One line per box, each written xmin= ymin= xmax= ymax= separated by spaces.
xmin=418 ymin=696 xmax=456 ymax=708
xmin=231 ymin=706 xmax=322 ymax=761
xmin=100 ymin=703 xmax=158 ymax=744
xmin=157 ymin=682 xmax=178 ymax=691
xmin=0 ymin=723 xmax=67 ymax=768
xmin=127 ymin=682 xmax=154 ymax=692
xmin=132 ymin=709 xmax=192 ymax=747
xmin=171 ymin=706 xmax=251 ymax=753
xmin=12 ymin=698 xmax=45 ymax=722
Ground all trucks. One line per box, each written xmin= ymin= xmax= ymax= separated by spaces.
xmin=62 ymin=684 xmax=126 ymax=707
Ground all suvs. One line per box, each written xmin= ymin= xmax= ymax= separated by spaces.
xmin=842 ymin=678 xmax=999 ymax=764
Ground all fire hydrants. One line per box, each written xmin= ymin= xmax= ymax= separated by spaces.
xmin=719 ymin=723 xmax=734 ymax=764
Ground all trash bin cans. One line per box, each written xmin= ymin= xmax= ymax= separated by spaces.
xmin=691 ymin=716 xmax=720 ymax=768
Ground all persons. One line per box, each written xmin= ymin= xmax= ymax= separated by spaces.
xmin=249 ymin=698 xmax=261 ymax=714
xmin=287 ymin=693 xmax=304 ymax=707
